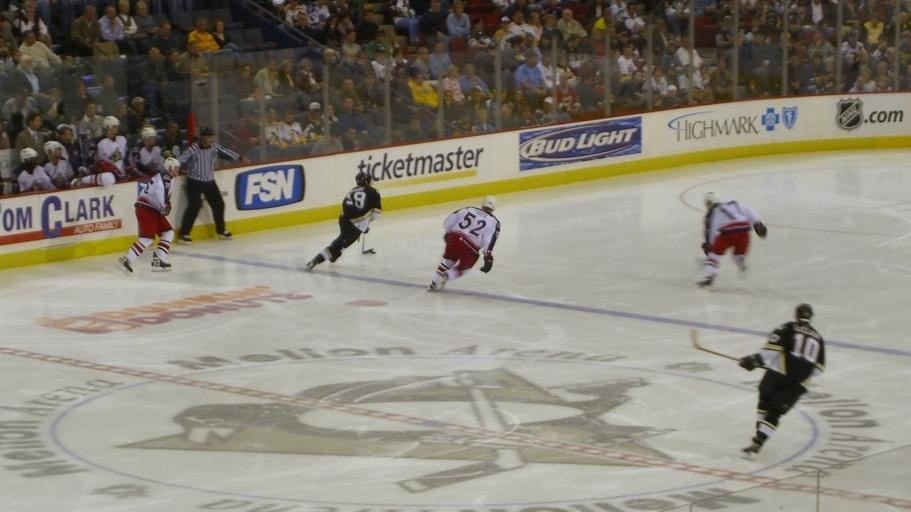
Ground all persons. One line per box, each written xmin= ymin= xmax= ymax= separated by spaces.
xmin=0 ymin=0 xmax=181 ymax=190
xmin=738 ymin=302 xmax=825 ymax=457
xmin=117 ymin=157 xmax=181 ymax=273
xmin=303 ymin=173 xmax=383 ymax=273
xmin=390 ymin=1 xmax=660 ymax=139
xmin=694 ymin=190 xmax=768 ymax=288
xmin=176 ymin=123 xmax=252 ymax=244
xmin=425 ymin=194 xmax=500 ymax=294
xmin=659 ymin=0 xmax=911 ymax=111
xmin=70 ymin=1 xmax=436 ymax=153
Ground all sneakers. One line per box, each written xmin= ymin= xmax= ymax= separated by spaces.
xmin=217 ymin=229 xmax=232 ymax=237
xmin=118 ymin=255 xmax=133 ymax=273
xmin=178 ymin=233 xmax=193 ymax=241
xmin=436 ymin=272 xmax=448 ymax=290
xmin=696 ymin=273 xmax=717 ymax=286
xmin=743 ymin=442 xmax=760 ymax=453
xmin=151 ymin=253 xmax=171 ymax=267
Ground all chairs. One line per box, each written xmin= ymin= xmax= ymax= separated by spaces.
xmin=1 ymin=0 xmax=722 ymax=198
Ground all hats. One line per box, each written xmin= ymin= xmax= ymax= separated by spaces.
xmin=200 ymin=125 xmax=218 ymax=137
xmin=309 ymin=102 xmax=322 ymax=113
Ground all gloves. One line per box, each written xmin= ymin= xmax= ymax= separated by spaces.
xmin=753 ymin=221 xmax=767 ymax=236
xmin=738 ymin=353 xmax=763 ymax=371
xmin=480 ymin=250 xmax=493 ymax=273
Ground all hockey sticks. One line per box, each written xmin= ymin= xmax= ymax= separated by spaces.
xmin=362 ymin=234 xmax=374 ymax=254
xmin=690 ymin=328 xmax=768 ymax=369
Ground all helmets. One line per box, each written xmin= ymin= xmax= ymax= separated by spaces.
xmin=355 ymin=172 xmax=371 ymax=187
xmin=163 ymin=157 xmax=180 ymax=172
xmin=103 ymin=116 xmax=120 ymax=130
xmin=141 ymin=127 xmax=156 ymax=142
xmin=43 ymin=140 xmax=62 ymax=155
xmin=797 ymin=304 xmax=812 ymax=323
xmin=703 ymin=191 xmax=722 ymax=206
xmin=481 ymin=194 xmax=496 ymax=211
xmin=20 ymin=147 xmax=37 ymax=164
